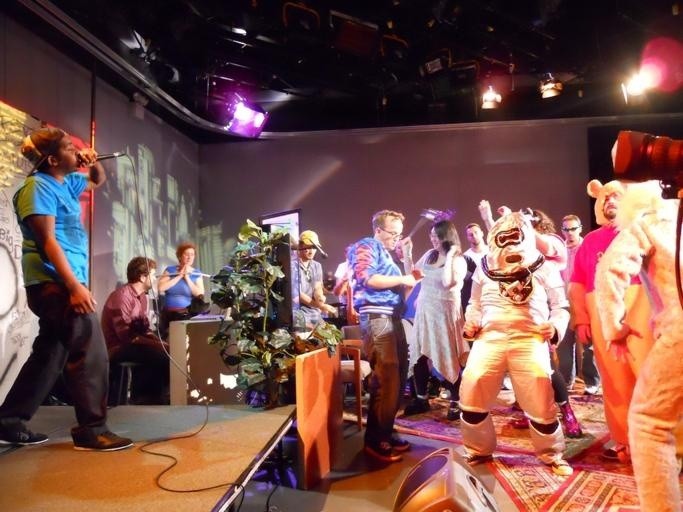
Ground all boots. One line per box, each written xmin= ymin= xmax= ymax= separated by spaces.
xmin=558 ymin=401 xmax=582 ymax=438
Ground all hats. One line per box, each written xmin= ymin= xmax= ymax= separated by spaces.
xmin=299 ymin=230 xmax=320 ymax=246
xmin=18 ymin=127 xmax=68 ymax=177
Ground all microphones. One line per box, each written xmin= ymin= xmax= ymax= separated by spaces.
xmin=80 ymin=150 xmax=125 ymax=164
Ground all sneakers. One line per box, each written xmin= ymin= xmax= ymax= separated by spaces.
xmin=0 ymin=419 xmax=49 ymax=445
xmin=551 ymin=459 xmax=573 ymax=476
xmin=404 ymin=396 xmax=431 ymax=416
xmin=363 ymin=435 xmax=403 ymax=463
xmin=467 ymin=453 xmax=494 ymax=466
xmin=386 ymin=435 xmax=411 ymax=452
xmin=446 ymin=397 xmax=460 ymax=420
xmin=70 ymin=425 xmax=134 ymax=452
xmin=601 ymin=442 xmax=630 ymax=459
xmin=584 ymin=383 xmax=600 ymax=395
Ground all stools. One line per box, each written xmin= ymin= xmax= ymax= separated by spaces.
xmin=114 ymin=354 xmax=141 ymax=405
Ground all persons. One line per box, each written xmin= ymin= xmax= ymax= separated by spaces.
xmin=0 ymin=127 xmax=134 ymax=451
xmin=158 ymin=243 xmax=205 ymax=342
xmin=100 ymin=257 xmax=169 ymax=405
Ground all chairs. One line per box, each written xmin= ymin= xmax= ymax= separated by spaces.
xmin=334 ymin=337 xmax=369 ymax=433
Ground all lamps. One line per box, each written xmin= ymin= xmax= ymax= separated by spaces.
xmin=482 ymin=77 xmax=502 ymax=111
xmin=529 ymin=68 xmax=563 ymax=97
xmin=223 ymin=89 xmax=269 ymax=140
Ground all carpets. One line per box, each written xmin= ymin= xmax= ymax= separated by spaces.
xmin=537 ymin=465 xmax=683 ymax=511
xmin=483 ymin=434 xmax=683 ymax=511
xmin=340 ymin=386 xmax=611 ymax=461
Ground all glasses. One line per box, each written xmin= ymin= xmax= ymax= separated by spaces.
xmin=561 ymin=224 xmax=581 ymax=232
xmin=380 ymin=226 xmax=402 ymax=238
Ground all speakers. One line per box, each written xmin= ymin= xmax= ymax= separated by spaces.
xmin=391 ymin=446 xmax=500 ymax=512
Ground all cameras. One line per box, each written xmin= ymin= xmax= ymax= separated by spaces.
xmin=613 ymin=130 xmax=683 ymax=199
